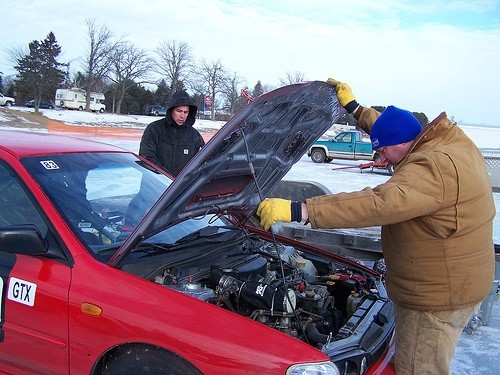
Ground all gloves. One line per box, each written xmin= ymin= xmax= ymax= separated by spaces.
xmin=256 ymin=197 xmax=302 ymax=232
xmin=326 ymin=77 xmax=359 ymax=115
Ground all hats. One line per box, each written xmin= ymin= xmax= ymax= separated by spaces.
xmin=370 ymin=105 xmax=423 ymax=149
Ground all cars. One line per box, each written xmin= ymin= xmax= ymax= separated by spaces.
xmin=0 ymin=93 xmax=16 ymax=107
xmin=0 ymin=80 xmax=400 ymax=374
xmin=144 ymin=104 xmax=168 ymax=116
xmin=25 ymin=98 xmax=55 ymax=109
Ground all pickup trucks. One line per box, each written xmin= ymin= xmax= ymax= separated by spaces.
xmin=307 ymin=131 xmax=380 ymax=164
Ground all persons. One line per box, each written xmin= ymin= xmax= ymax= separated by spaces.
xmin=257 ymin=77 xmax=495 ymax=375
xmin=139 ymin=92 xmax=206 ymax=208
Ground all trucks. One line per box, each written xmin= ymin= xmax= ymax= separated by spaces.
xmin=55 ymin=87 xmax=107 ymax=113
